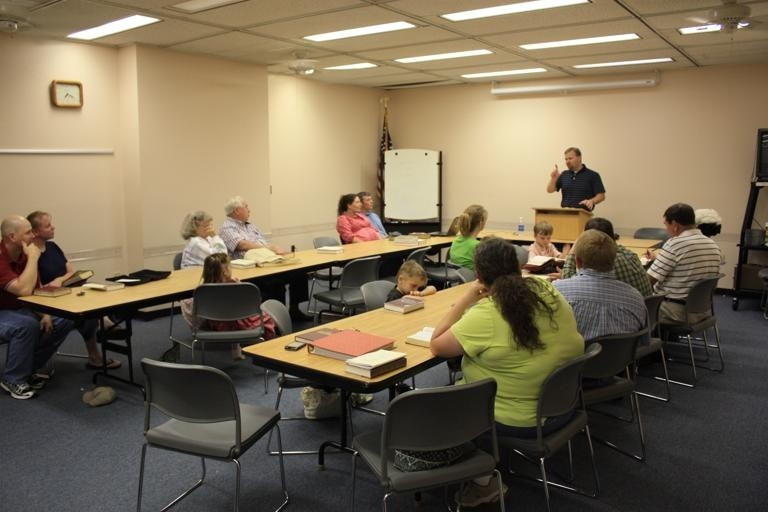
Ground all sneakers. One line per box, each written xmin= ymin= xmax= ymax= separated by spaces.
xmin=232 ymin=349 xmax=245 ymax=361
xmin=26 ymin=376 xmax=45 ymax=389
xmin=454 ymin=475 xmax=510 ymax=509
xmin=0 ymin=379 xmax=36 ymax=400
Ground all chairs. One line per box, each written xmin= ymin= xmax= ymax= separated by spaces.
xmin=635 ymin=272 xmax=726 ymax=390
xmin=560 ymin=325 xmax=649 ymax=486
xmin=634 ymin=227 xmax=669 ymax=242
xmin=422 ymin=247 xmax=464 ymax=290
xmin=307 ymin=238 xmax=346 ymax=317
xmin=191 ymin=282 xmax=270 ymax=395
xmin=351 ymin=377 xmax=504 ymax=512
xmin=312 ymin=255 xmax=382 ymax=328
xmin=500 ymin=343 xmax=600 ymax=511
xmin=260 ymin=298 xmax=354 ymax=455
xmin=585 ymin=289 xmax=674 ymax=425
xmin=169 ymin=252 xmax=193 ymax=350
xmin=383 ymin=246 xmax=431 ymax=285
xmin=360 ymin=280 xmax=419 ymax=390
xmin=136 ymin=357 xmax=288 ymax=512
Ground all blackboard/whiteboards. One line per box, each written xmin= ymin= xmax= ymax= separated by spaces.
xmin=383 ymin=148 xmax=439 ymax=220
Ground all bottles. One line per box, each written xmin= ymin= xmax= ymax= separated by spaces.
xmin=517 ymin=217 xmax=525 ymax=237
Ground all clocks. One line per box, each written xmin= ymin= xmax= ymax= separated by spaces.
xmin=51 ymin=79 xmax=83 ymax=108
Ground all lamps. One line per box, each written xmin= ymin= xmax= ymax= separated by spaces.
xmin=574 ymin=57 xmax=673 ymax=69
xmin=519 ymin=32 xmax=643 ymax=51
xmin=395 ymin=49 xmax=494 ymax=64
xmin=324 ymin=62 xmax=378 ymax=71
xmin=302 ymin=21 xmax=416 ymax=42
xmin=440 ymin=0 xmax=590 ymax=22
xmin=679 ymin=23 xmax=750 ymax=35
xmin=67 ymin=14 xmax=161 ymax=41
xmin=461 ymin=67 xmax=548 ymax=78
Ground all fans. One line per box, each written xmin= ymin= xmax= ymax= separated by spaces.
xmin=273 ymin=45 xmax=321 ymax=75
xmin=0 ymin=0 xmax=37 ymax=35
xmin=684 ymin=0 xmax=768 ymax=32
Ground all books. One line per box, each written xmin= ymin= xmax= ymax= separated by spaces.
xmin=229 ymin=248 xmax=282 ymax=271
xmin=319 ymin=245 xmax=344 ymax=254
xmin=294 ymin=327 xmax=338 ymax=346
xmin=61 ymin=269 xmax=93 ymax=287
xmin=383 ymin=296 xmax=424 ymax=314
xmin=525 ymin=254 xmax=567 ymax=267
xmin=33 ymin=282 xmax=72 ymax=298
xmin=344 ymin=347 xmax=407 ymax=379
xmin=405 ymin=326 xmax=435 ymax=348
xmin=82 ymin=280 xmax=125 ymax=292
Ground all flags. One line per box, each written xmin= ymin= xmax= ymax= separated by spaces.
xmin=376 ymin=97 xmax=394 ymax=202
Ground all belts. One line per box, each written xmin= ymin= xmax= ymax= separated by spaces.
xmin=662 ymin=297 xmax=686 ymax=306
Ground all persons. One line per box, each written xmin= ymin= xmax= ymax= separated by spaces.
xmin=219 ymin=196 xmax=315 ymax=321
xmin=203 ymin=253 xmax=276 ymax=341
xmin=547 ymin=217 xmax=652 ymax=297
xmin=337 ymin=193 xmax=382 ymax=243
xmin=527 ymin=222 xmax=570 ymax=263
xmin=647 ymin=203 xmax=722 ymax=325
xmin=546 ymin=147 xmax=606 ymax=253
xmin=551 ymin=229 xmax=647 ymax=389
xmin=428 ymin=235 xmax=585 ymax=508
xmin=642 ymin=248 xmax=661 ymax=260
xmin=27 ymin=211 xmax=129 ymax=370
xmin=358 ymin=191 xmax=388 ymax=235
xmin=180 ymin=211 xmax=227 ymax=326
xmin=384 ymin=260 xmax=437 ymax=301
xmin=0 ymin=213 xmax=70 ymax=400
xmin=449 ymin=204 xmax=487 ymax=271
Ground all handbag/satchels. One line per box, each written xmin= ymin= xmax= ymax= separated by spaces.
xmin=301 ymin=387 xmax=349 ymax=419
xmin=386 ymin=383 xmax=476 ymax=472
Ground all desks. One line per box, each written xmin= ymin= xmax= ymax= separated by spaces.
xmin=241 ymin=246 xmax=652 ymax=509
xmin=14 ymin=236 xmax=462 ymax=406
xmin=476 ymin=206 xmax=664 ymax=249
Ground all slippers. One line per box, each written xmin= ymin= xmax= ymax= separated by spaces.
xmin=97 ymin=325 xmax=126 ymax=343
xmin=85 ymin=357 xmax=121 ymax=371
